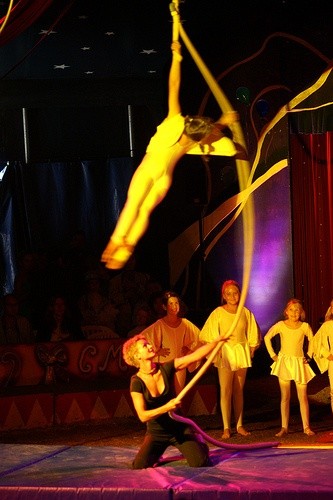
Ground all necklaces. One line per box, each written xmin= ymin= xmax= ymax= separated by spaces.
xmin=139 ymin=363 xmax=157 ymax=375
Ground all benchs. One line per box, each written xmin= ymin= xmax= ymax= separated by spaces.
xmin=0 ymin=338 xmax=216 ymax=437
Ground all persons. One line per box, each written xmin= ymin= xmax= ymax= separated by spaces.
xmin=0 ymin=253 xmax=166 ymax=346
xmin=122 ymin=334 xmax=235 ymax=469
xmin=312 ymin=297 xmax=333 ymax=413
xmin=199 ymin=280 xmax=261 ymax=439
xmin=102 ymin=41 xmax=238 ymax=269
xmin=139 ymin=293 xmax=210 ymax=398
xmin=263 ymin=298 xmax=317 ymax=437
